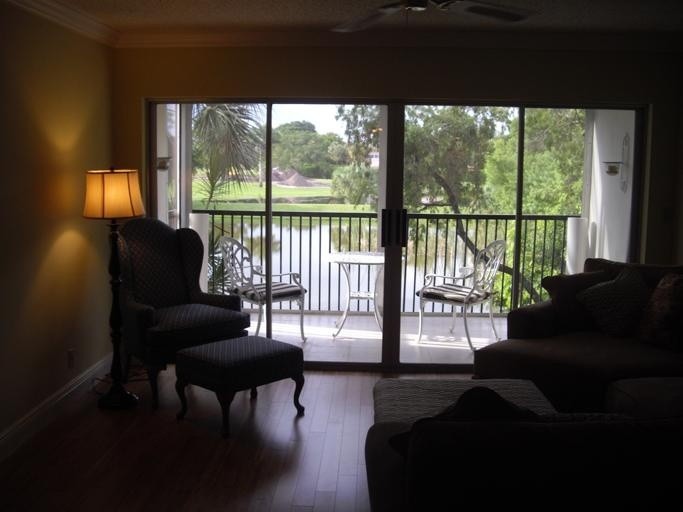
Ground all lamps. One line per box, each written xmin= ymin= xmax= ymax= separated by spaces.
xmin=80 ymin=163 xmax=147 ymax=411
xmin=602 ymin=131 xmax=630 ymax=195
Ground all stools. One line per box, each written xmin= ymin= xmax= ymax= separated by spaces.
xmin=605 ymin=376 xmax=682 ymax=419
xmin=173 ymin=335 xmax=306 ymax=433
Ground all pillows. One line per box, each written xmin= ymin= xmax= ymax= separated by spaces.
xmin=574 ymin=262 xmax=647 ymax=341
xmin=539 ymin=270 xmax=610 ymax=335
xmin=648 ymin=268 xmax=682 ymax=343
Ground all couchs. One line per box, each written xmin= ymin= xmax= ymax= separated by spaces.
xmin=364 ymin=386 xmax=682 ymax=512
xmin=472 ymin=252 xmax=682 ymax=410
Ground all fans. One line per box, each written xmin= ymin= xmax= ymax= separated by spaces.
xmin=329 ymin=2 xmax=536 ymax=35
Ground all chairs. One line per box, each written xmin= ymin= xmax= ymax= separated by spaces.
xmin=416 ymin=238 xmax=508 ymax=353
xmin=114 ymin=214 xmax=251 ymax=406
xmin=218 ymin=234 xmax=310 ymax=340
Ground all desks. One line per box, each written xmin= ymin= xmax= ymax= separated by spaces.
xmin=327 ymin=250 xmax=405 ymax=339
